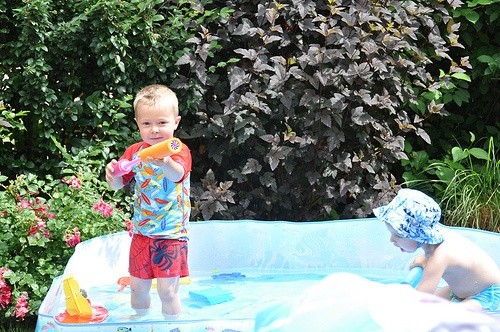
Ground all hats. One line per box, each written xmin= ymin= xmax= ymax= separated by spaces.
xmin=373 ymin=188 xmax=444 ymax=245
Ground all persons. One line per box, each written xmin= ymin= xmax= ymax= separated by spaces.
xmin=374 ymin=187 xmax=499 ymax=312
xmin=105 ymin=85 xmax=192 ymax=316
